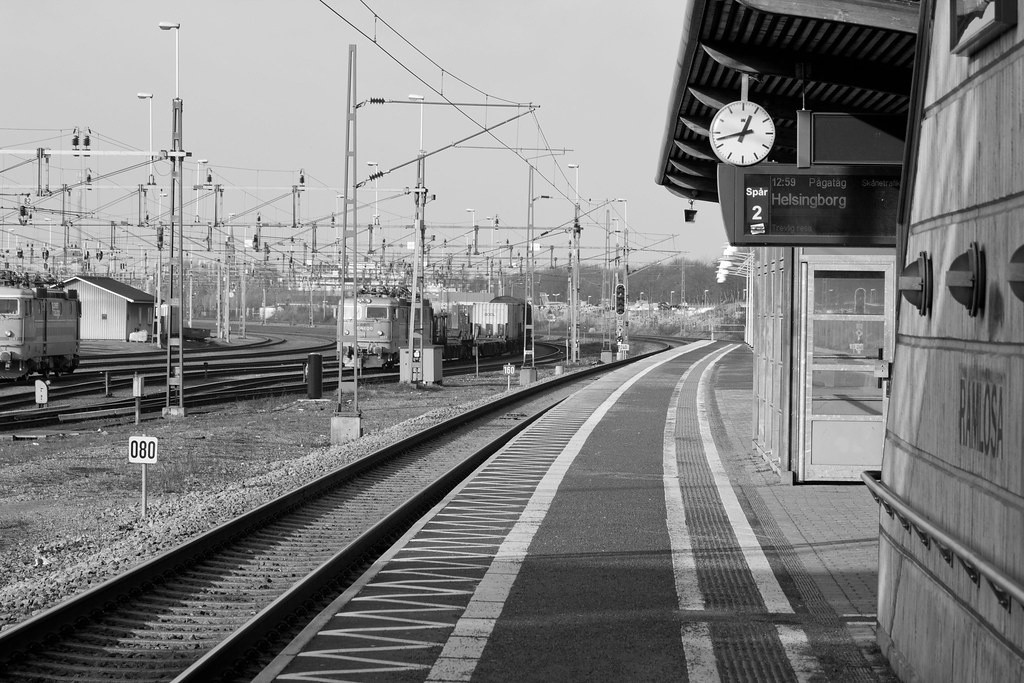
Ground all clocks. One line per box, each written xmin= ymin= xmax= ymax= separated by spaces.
xmin=709 ymin=100 xmax=776 ymax=166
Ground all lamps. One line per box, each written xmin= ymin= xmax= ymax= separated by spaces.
xmin=684 ymin=199 xmax=697 ymax=222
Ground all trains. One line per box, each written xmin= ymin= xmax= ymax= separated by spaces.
xmin=336 ymin=285 xmax=449 ymax=368
xmin=0 ymin=280 xmax=84 ymax=384
xmin=447 ymin=295 xmax=533 ymax=360
